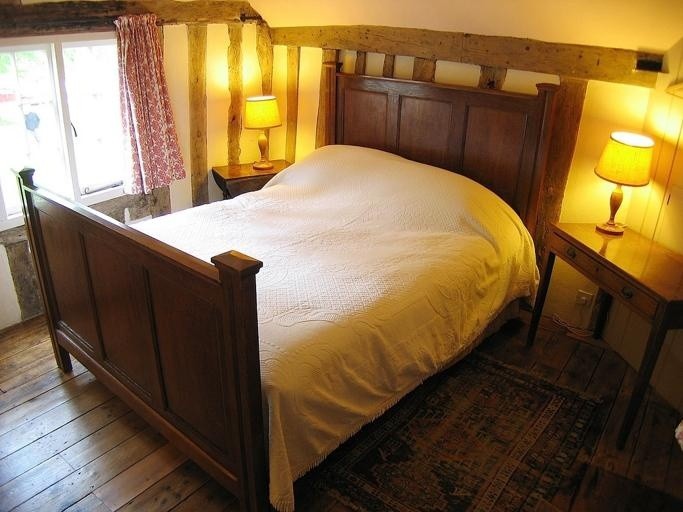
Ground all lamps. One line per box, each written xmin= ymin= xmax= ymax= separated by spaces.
xmin=243 ymin=94 xmax=282 ymax=170
xmin=594 ymin=131 xmax=655 ymax=236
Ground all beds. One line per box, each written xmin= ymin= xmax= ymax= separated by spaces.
xmin=16 ymin=62 xmax=563 ymax=512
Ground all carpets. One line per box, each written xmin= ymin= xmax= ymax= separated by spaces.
xmin=307 ymin=347 xmax=601 ymax=511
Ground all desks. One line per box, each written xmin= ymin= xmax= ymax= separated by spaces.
xmin=528 ymin=222 xmax=682 ymax=450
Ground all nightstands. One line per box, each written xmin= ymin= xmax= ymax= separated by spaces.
xmin=212 ymin=160 xmax=290 ymax=200
